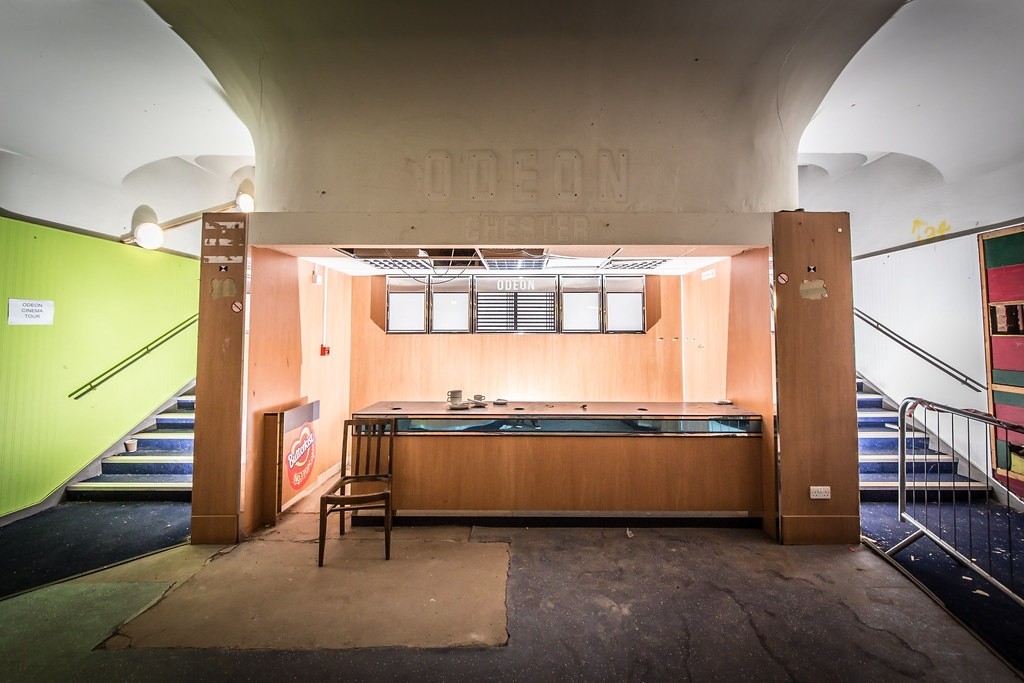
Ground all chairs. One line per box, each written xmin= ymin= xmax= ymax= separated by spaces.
xmin=318 ymin=417 xmax=395 ymax=567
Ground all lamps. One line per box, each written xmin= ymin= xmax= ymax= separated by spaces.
xmin=120 ymin=193 xmax=254 ymax=251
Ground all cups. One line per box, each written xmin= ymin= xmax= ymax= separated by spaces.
xmin=474 ymin=395 xmax=485 ymax=401
xmin=447 ymin=390 xmax=462 ymax=397
xmin=447 ymin=397 xmax=462 ymax=405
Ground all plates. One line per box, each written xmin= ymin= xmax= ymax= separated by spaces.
xmin=449 ymin=405 xmax=468 ymax=409
xmin=493 ymin=401 xmax=507 ymax=404
